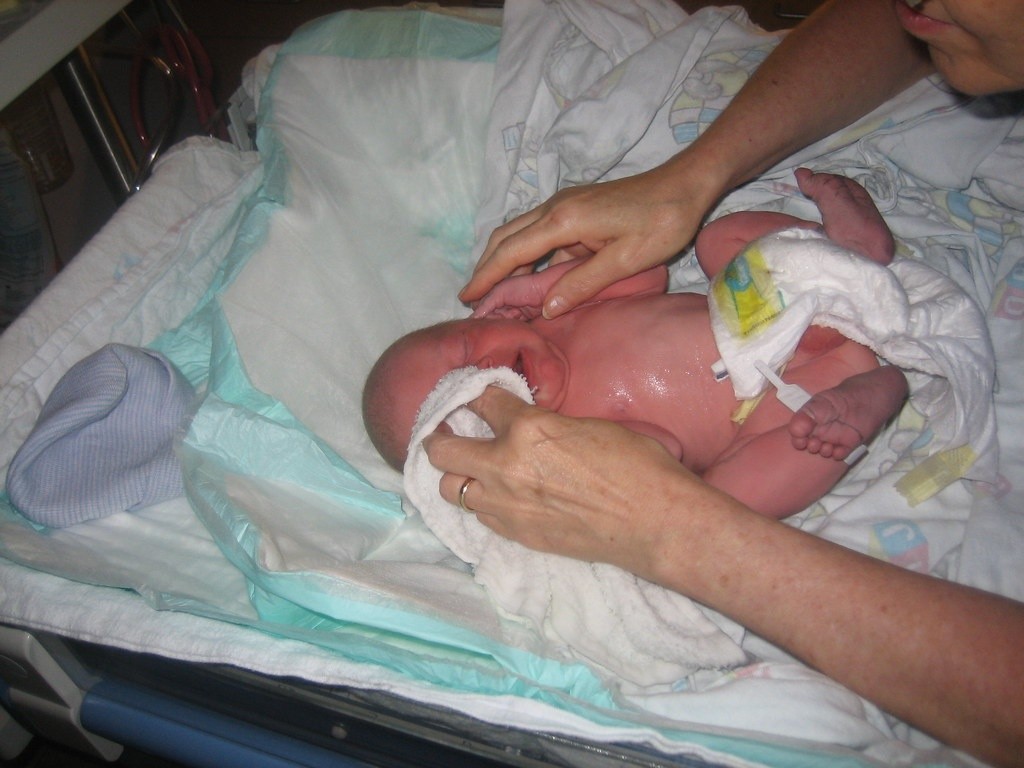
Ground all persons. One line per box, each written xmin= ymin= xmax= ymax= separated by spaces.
xmin=361 ymin=166 xmax=910 ymax=521
xmin=423 ymin=0 xmax=1024 ymax=767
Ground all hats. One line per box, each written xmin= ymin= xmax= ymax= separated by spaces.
xmin=6 ymin=343 xmax=198 ymax=529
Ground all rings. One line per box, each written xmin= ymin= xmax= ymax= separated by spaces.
xmin=459 ymin=478 xmax=476 ymax=513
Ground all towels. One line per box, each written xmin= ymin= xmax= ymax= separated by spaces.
xmin=403 ymin=361 xmax=744 ymax=676
